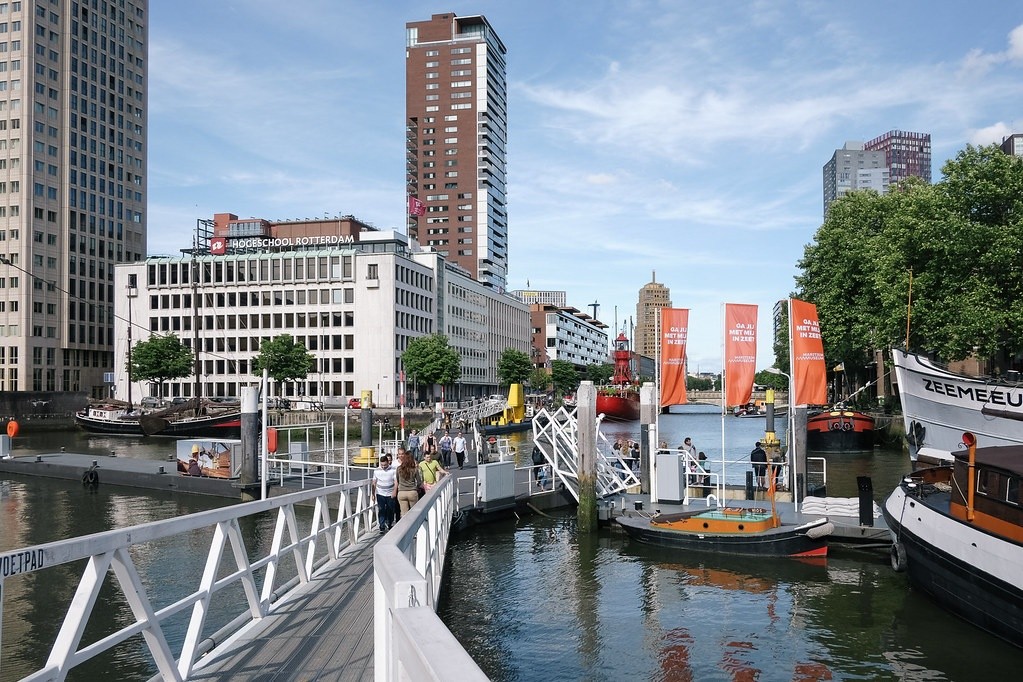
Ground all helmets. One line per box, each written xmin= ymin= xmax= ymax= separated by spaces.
xmin=200 ymin=447 xmax=205 ymax=452
xmin=191 ymin=444 xmax=198 ymax=454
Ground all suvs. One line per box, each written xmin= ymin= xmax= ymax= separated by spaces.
xmin=141 ymin=397 xmax=190 ymax=409
xmin=489 ymin=395 xmax=507 ymax=402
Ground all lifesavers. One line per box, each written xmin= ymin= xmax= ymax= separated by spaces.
xmin=7 ymin=426 xmax=16 ymax=437
xmin=891 ymin=542 xmax=908 ymax=573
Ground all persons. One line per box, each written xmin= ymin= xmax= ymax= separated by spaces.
xmin=612 ymin=437 xmax=706 ymax=485
xmin=470 ymin=394 xmax=488 ymax=406
xmin=445 ymin=414 xmax=468 ymax=434
xmin=371 ymin=429 xmax=468 ymax=535
xmin=751 ymin=441 xmax=782 ymax=489
xmin=532 ymin=444 xmax=549 ymax=492
xmin=384 ymin=417 xmax=391 ymax=432
xmin=188 ymin=459 xmax=202 ymax=476
xmin=192 ymin=444 xmax=205 ymax=468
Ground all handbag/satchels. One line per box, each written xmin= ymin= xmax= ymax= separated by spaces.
xmin=538 ymin=468 xmax=544 ymax=478
xmin=464 ymin=449 xmax=469 ymax=464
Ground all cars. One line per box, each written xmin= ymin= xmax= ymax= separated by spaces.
xmin=349 ymin=398 xmax=376 ymax=409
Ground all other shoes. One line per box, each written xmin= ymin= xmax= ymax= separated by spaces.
xmin=379 ymin=531 xmax=384 ymax=535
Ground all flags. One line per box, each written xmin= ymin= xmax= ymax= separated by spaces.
xmin=659 ymin=307 xmax=689 ymax=408
xmin=789 ymin=298 xmax=828 ymax=406
xmin=725 ymin=303 xmax=758 ymax=407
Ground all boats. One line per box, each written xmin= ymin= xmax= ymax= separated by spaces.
xmin=618 ymin=535 xmax=833 ymax=594
xmin=596 ymin=333 xmax=640 ymax=419
xmin=734 ymin=400 xmax=787 ymax=418
xmin=615 ymin=441 xmax=835 ymax=557
xmin=73 ymin=236 xmax=263 ymax=438
xmin=807 ymin=349 xmax=1023 ymax=650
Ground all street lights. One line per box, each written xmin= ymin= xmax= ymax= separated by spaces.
xmin=764 ymin=368 xmax=790 ymax=492
